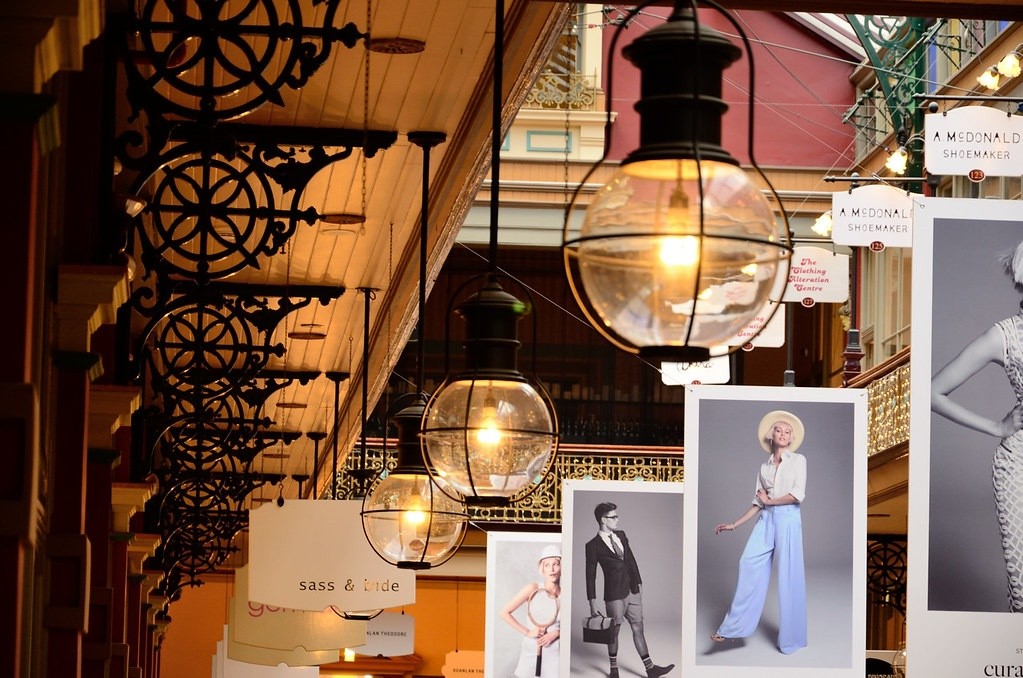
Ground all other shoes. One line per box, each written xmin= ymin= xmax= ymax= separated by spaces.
xmin=711 ymin=634 xmax=728 ymax=641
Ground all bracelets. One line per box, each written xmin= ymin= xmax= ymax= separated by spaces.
xmin=729 ymin=522 xmax=735 ymax=532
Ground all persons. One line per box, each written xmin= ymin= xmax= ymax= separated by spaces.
xmin=500 ymin=542 xmax=562 ymax=676
xmin=707 ymin=407 xmax=810 ymax=653
xmin=583 ymin=500 xmax=676 ymax=676
xmin=928 ymin=239 xmax=1019 ymax=620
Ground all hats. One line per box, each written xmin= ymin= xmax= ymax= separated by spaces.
xmin=759 ymin=410 xmax=805 ymax=454
xmin=537 ymin=546 xmax=562 ymax=562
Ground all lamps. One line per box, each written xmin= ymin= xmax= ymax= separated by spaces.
xmin=358 ymin=387 xmax=472 ymax=571
xmin=977 ymin=64 xmax=1004 ymax=93
xmin=997 ymin=42 xmax=1022 ymax=78
xmin=886 ymin=132 xmax=925 ymax=174
xmin=810 ymin=209 xmax=834 ymax=239
xmin=415 ymin=270 xmax=565 ymax=508
xmin=559 ymin=1 xmax=793 ymax=363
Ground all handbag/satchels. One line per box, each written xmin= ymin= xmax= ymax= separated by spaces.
xmin=582 ymin=611 xmax=615 ymax=644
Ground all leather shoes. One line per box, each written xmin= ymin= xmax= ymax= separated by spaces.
xmin=647 ymin=664 xmax=674 ymax=678
